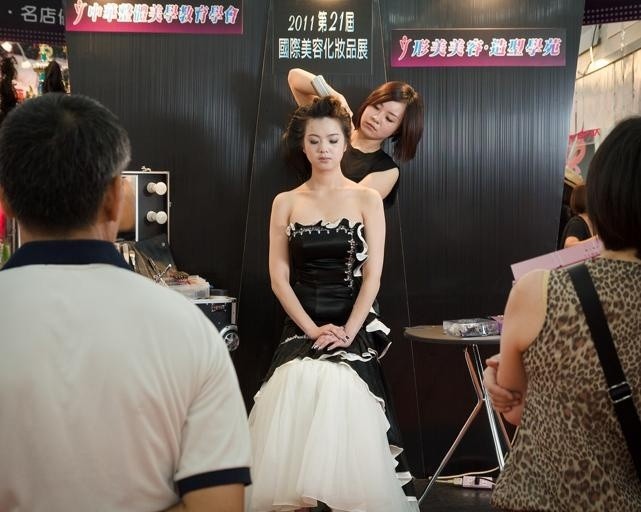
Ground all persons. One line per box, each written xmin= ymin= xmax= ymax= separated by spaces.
xmin=43 ymin=60 xmax=66 ymax=96
xmin=482 ymin=113 xmax=641 ymax=512
xmin=247 ymin=94 xmax=425 ymax=512
xmin=560 ymin=182 xmax=598 ymax=252
xmin=1 ymin=54 xmax=18 ymax=119
xmin=1 ymin=91 xmax=254 ymax=512
xmin=285 ymin=67 xmax=425 ymax=205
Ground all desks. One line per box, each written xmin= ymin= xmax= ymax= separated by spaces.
xmin=403 ymin=324 xmax=512 ymax=507
